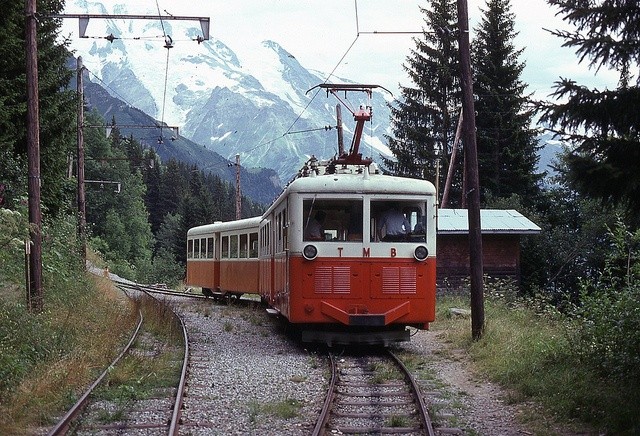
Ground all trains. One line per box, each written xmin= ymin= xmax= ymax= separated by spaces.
xmin=186 ymin=161 xmax=437 ymax=347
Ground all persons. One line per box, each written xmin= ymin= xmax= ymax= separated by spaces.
xmin=104 ymin=266 xmax=110 ymax=278
xmin=377 ymin=209 xmax=411 ymax=243
xmin=306 ymin=212 xmax=326 ymax=243
